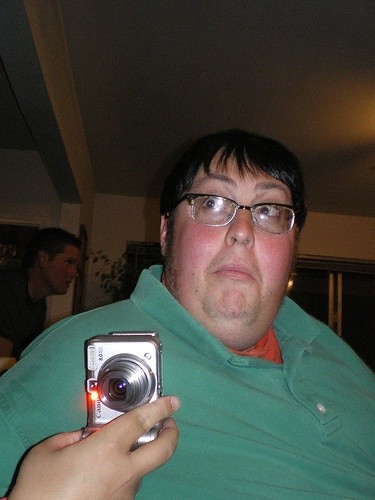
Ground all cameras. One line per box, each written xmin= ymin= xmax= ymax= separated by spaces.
xmin=83 ymin=331 xmax=165 ymax=453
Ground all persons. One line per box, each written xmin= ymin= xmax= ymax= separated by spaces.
xmin=0 ymin=227 xmax=81 ymax=373
xmin=0 ymin=126 xmax=375 ymax=500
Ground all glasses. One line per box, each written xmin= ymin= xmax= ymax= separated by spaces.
xmin=164 ymin=190 xmax=299 ymax=238
